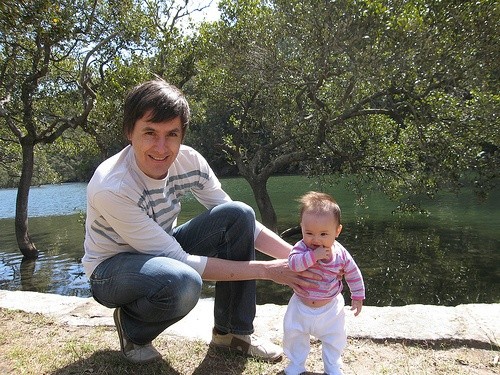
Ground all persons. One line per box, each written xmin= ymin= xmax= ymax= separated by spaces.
xmin=280 ymin=191 xmax=365 ymax=375
xmin=81 ymin=73 xmax=344 ymax=365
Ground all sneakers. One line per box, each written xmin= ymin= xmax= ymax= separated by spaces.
xmin=212 ymin=328 xmax=283 ymax=361
xmin=113 ymin=307 xmax=163 ymax=364
